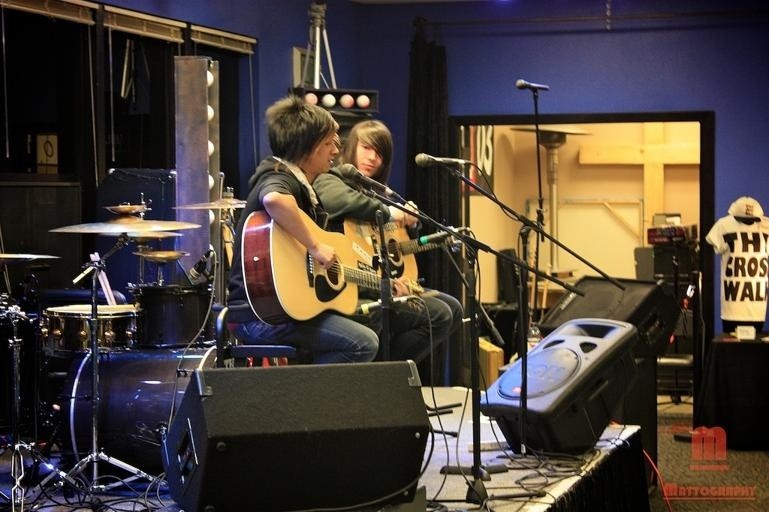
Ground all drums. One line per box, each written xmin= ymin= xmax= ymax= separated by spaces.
xmin=70 ymin=350 xmax=222 ymax=496
xmin=127 ymin=283 xmax=217 ymax=348
xmin=42 ymin=304 xmax=141 ymax=357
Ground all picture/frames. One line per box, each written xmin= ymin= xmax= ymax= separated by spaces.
xmin=290 ymin=45 xmax=316 ymax=89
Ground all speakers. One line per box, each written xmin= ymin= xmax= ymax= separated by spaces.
xmin=479 ymin=316 xmax=637 ymax=455
xmin=164 ymin=359 xmax=428 ymax=512
xmin=538 ymin=274 xmax=682 ymax=356
xmin=609 ymin=351 xmax=658 ymax=495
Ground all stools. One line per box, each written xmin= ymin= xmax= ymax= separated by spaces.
xmin=225 ymin=341 xmax=299 ymax=368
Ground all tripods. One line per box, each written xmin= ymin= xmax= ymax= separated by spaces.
xmin=300 ymin=3 xmax=337 ymax=89
xmin=452 ymin=170 xmax=580 ymax=465
xmin=0 ymin=320 xmax=107 ymax=512
xmin=31 ymin=272 xmax=170 ymax=512
xmin=393 ymin=192 xmax=584 ymax=512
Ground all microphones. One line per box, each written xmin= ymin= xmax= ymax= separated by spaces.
xmin=189 ymin=251 xmax=209 ymax=280
xmin=515 ymin=79 xmax=549 ymax=90
xmin=341 ymin=163 xmax=396 ymax=198
xmin=415 ymin=153 xmax=474 ymax=167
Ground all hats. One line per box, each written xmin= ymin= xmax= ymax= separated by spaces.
xmin=728 ymin=195 xmax=764 ymax=222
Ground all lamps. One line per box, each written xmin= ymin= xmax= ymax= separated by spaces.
xmin=173 ymin=56 xmax=223 ymax=282
xmin=293 ymin=84 xmax=382 ymax=118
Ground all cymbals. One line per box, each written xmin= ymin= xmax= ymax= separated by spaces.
xmin=102 ymin=234 xmax=183 ymax=238
xmin=49 ymin=217 xmax=202 ymax=233
xmin=172 ymin=198 xmax=246 ymax=209
xmin=1 ymin=254 xmax=61 ymax=260
xmin=133 ymin=251 xmax=190 ymax=261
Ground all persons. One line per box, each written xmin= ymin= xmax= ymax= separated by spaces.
xmin=704 ymin=195 xmax=768 ymax=341
xmin=227 ymin=96 xmax=409 ymax=364
xmin=313 ymin=120 xmax=463 ymax=363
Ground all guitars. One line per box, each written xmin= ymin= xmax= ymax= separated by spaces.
xmin=241 ymin=208 xmax=424 ymax=325
xmin=327 ymin=217 xmax=462 ymax=285
